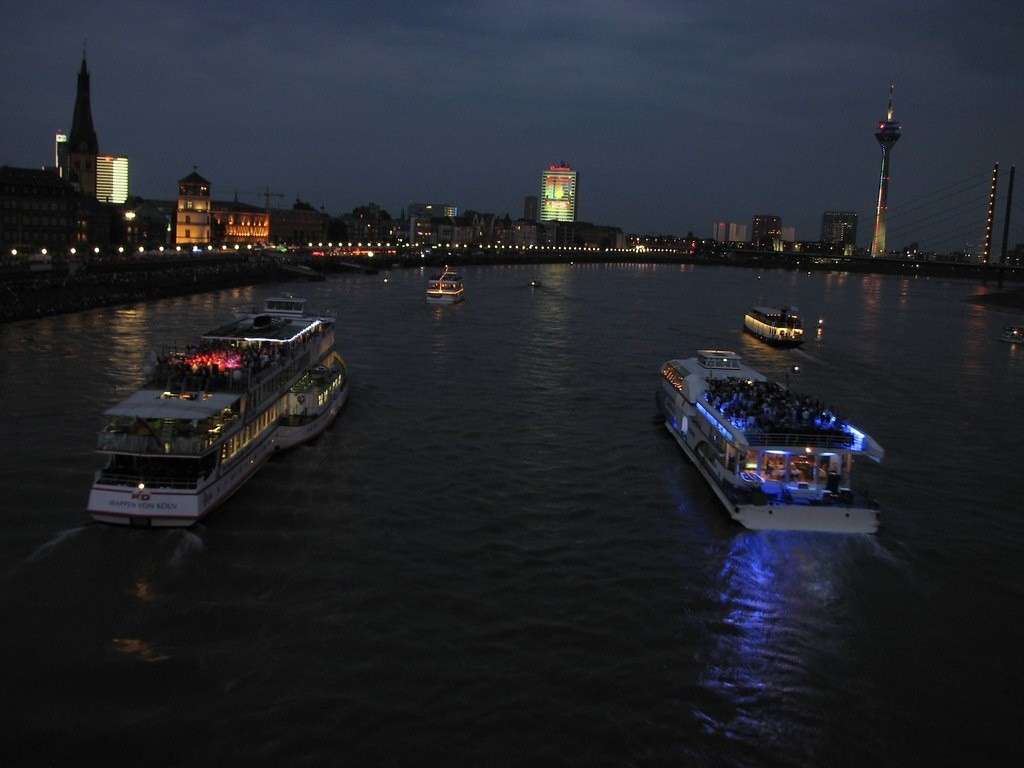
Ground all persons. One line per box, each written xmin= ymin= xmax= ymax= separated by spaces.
xmin=130 ymin=324 xmax=337 ymax=445
xmin=706 ymin=376 xmax=846 ymax=477
xmin=431 ymin=274 xmax=463 ymax=291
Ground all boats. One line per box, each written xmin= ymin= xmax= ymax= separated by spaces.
xmin=655 ymin=348 xmax=884 ymax=534
xmin=425 ymin=265 xmax=466 ymax=306
xmin=743 ymin=304 xmax=806 ymax=347
xmin=83 ymin=291 xmax=351 ymax=530
xmin=997 ymin=324 xmax=1024 ymax=344
